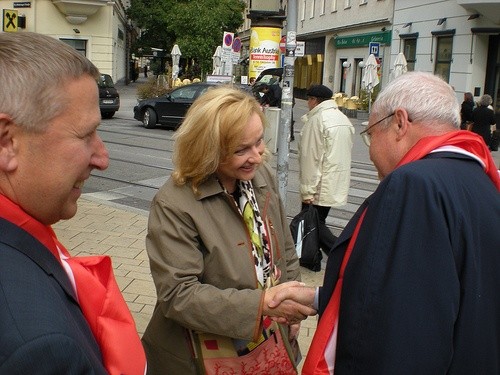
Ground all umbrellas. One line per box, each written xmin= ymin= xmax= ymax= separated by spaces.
xmin=171 ymin=45 xmax=408 ymax=126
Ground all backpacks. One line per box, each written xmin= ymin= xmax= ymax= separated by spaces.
xmin=290 ymin=205 xmax=321 ymax=264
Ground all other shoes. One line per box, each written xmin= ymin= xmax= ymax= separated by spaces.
xmin=300 ymin=261 xmax=321 ymax=272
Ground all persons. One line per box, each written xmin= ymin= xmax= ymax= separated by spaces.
xmin=248 ymin=77 xmax=295 ymax=142
xmin=268 ymin=72 xmax=500 ymax=375
xmin=0 ymin=30 xmax=146 ymax=375
xmin=297 ymin=83 xmax=355 ymax=272
xmin=461 ymin=92 xmax=500 ymax=152
xmin=142 ymin=86 xmax=316 ymax=375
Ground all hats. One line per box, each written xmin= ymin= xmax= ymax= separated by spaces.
xmin=305 ymin=85 xmax=333 ymax=99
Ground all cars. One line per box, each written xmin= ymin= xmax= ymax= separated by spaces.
xmin=133 ymin=82 xmax=254 ymax=129
xmin=97 ymin=73 xmax=120 ymax=118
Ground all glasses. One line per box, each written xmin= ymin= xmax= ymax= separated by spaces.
xmin=360 ymin=112 xmax=411 ymax=147
